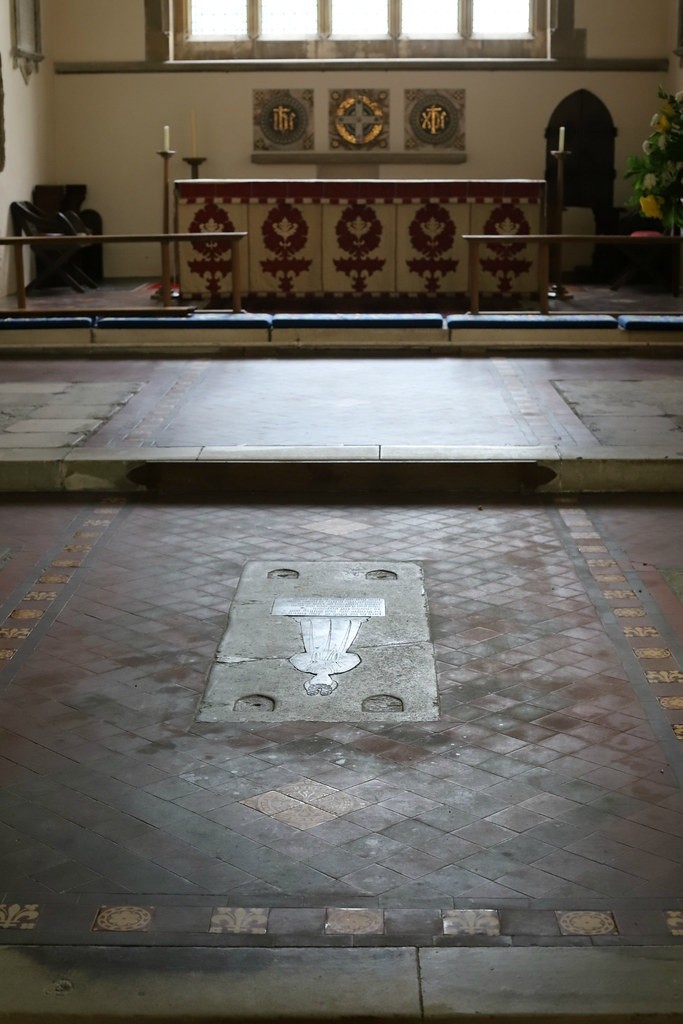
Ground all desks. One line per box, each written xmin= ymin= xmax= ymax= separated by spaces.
xmin=173 ymin=178 xmax=547 ymax=299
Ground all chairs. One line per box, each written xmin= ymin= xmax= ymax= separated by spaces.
xmin=10 ymin=202 xmax=99 ymax=293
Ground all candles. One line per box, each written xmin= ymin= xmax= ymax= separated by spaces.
xmin=191 ymin=111 xmax=196 ymax=158
xmin=163 ymin=126 xmax=169 ymax=151
xmin=559 ymin=127 xmax=565 ymax=152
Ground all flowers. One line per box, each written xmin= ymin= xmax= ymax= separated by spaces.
xmin=622 ymin=82 xmax=683 ymax=236
